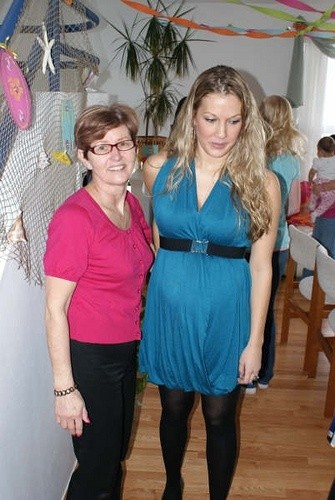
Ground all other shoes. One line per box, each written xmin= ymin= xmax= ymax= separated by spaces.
xmin=240 ymin=380 xmax=257 ymax=394
xmin=256 ymin=381 xmax=269 ymax=389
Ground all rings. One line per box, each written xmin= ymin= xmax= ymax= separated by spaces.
xmin=253 ymin=376 xmax=259 ymax=381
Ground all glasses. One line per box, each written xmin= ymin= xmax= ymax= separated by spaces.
xmin=87 ymin=139 xmax=135 ymax=156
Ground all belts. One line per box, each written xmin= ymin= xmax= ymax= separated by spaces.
xmin=159 ymin=234 xmax=246 ymax=259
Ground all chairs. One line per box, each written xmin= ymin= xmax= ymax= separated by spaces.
xmin=280 ymin=224 xmax=335 ymax=418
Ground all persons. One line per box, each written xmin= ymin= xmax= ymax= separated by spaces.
xmin=143 ymin=93 xmax=335 ymax=394
xmin=43 ymin=104 xmax=156 ymax=500
xmin=139 ymin=64 xmax=282 ymax=500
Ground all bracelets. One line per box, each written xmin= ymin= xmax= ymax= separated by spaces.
xmin=54 ymin=384 xmax=78 ymax=397
